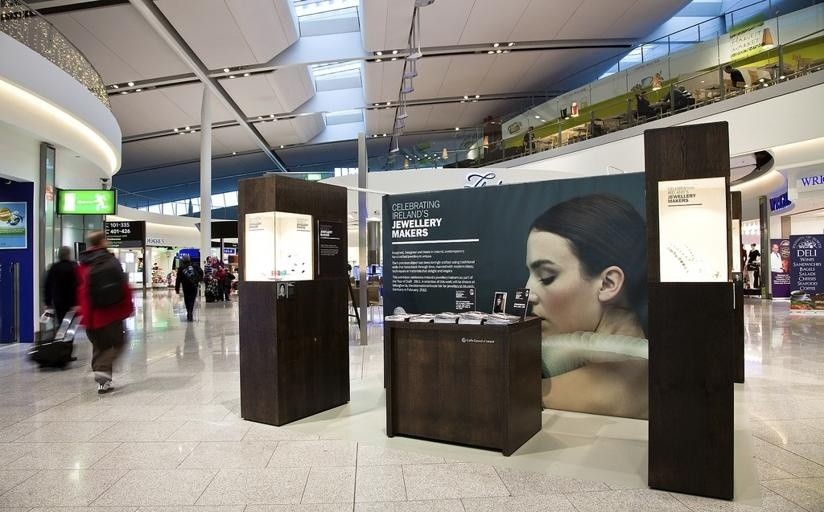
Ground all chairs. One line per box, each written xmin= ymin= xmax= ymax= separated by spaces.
xmin=351 ymin=287 xmax=383 ymax=325
xmin=521 ymin=52 xmax=816 ymax=152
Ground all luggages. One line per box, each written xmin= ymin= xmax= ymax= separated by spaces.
xmin=37 ymin=310 xmax=83 ymax=369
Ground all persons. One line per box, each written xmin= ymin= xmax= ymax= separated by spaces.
xmin=214 ymin=265 xmax=225 ymax=302
xmin=638 ymin=92 xmax=656 ymax=117
xmin=495 ymin=294 xmax=502 ymax=311
xmin=742 ymin=244 xmax=746 ymax=272
xmin=660 ymin=83 xmax=693 ymax=112
xmin=276 ymin=284 xmax=287 ymax=301
xmin=724 ymin=65 xmax=745 ymax=88
xmin=748 ymin=243 xmax=761 ymax=266
xmin=204 ymin=256 xmax=217 ymax=303
xmin=524 ymin=190 xmax=647 ymax=423
xmin=43 ymin=245 xmax=80 ymax=362
xmin=769 ymin=243 xmax=785 ymax=273
xmin=174 ymin=254 xmax=203 ymax=322
xmin=69 ymin=229 xmax=136 ymax=395
xmin=524 ymin=126 xmax=535 ymax=149
xmin=220 ymin=268 xmax=235 ymax=302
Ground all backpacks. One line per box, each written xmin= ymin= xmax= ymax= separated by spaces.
xmin=205 ymin=291 xmax=214 ymax=302
xmin=182 ymin=264 xmax=202 ymax=286
xmin=88 ymin=256 xmax=126 ymax=307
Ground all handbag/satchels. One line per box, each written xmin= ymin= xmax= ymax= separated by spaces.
xmin=39 ymin=308 xmax=61 ymax=340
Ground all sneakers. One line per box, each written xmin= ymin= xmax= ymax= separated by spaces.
xmin=187 ymin=312 xmax=193 ymax=321
xmin=94 ymin=371 xmax=116 ymax=394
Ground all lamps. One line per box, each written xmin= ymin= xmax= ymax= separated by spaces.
xmin=762 ymin=27 xmax=774 ymax=65
xmin=391 ymin=7 xmax=423 ymax=156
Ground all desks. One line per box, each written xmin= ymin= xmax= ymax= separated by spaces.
xmin=381 ymin=315 xmax=545 ymax=458
